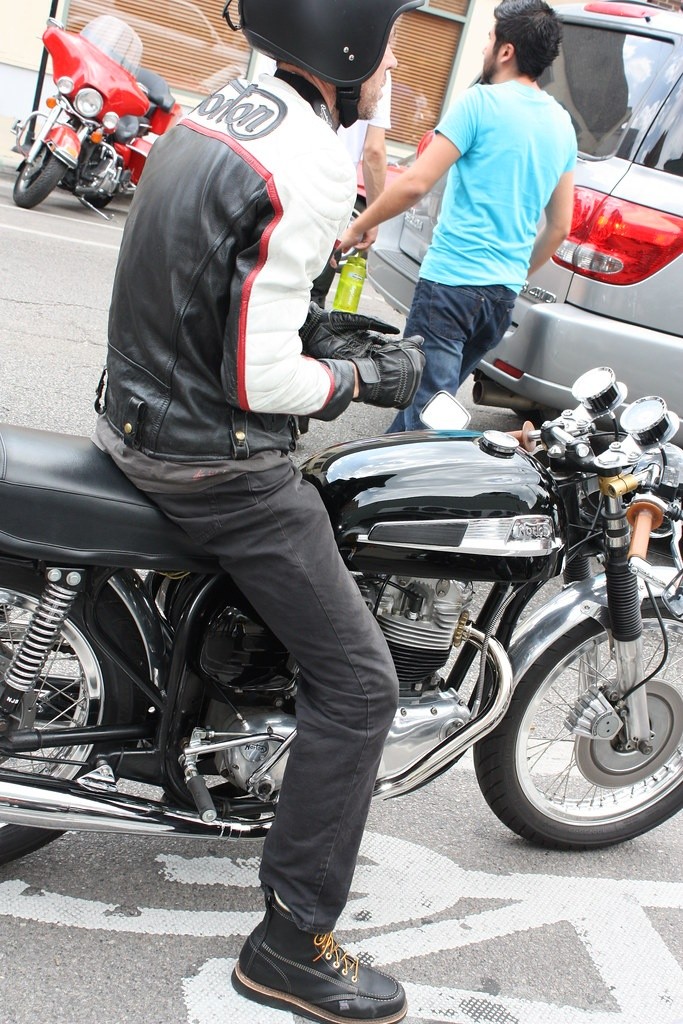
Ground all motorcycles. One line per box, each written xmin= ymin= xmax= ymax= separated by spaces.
xmin=0 ymin=365 xmax=682 ymax=865
xmin=10 ymin=14 xmax=185 ymax=220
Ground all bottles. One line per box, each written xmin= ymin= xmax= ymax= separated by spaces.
xmin=334 ymin=256 xmax=367 ymax=314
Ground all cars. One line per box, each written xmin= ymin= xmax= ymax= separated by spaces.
xmin=335 ymin=149 xmax=419 ymax=275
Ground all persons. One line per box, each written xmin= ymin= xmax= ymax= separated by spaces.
xmin=329 ymin=0 xmax=579 ymax=435
xmin=308 ymin=69 xmax=392 ymax=309
xmin=95 ymin=1 xmax=428 ymax=1023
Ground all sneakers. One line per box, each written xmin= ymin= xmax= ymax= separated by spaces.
xmin=230 ymin=883 xmax=408 ymax=1024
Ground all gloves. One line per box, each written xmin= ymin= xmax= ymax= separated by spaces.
xmin=297 ymin=301 xmax=427 ymax=411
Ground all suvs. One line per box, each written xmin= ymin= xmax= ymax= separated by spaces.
xmin=363 ymin=1 xmax=682 ymax=443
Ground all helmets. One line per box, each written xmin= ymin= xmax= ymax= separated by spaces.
xmin=221 ymin=0 xmax=427 ymax=88
